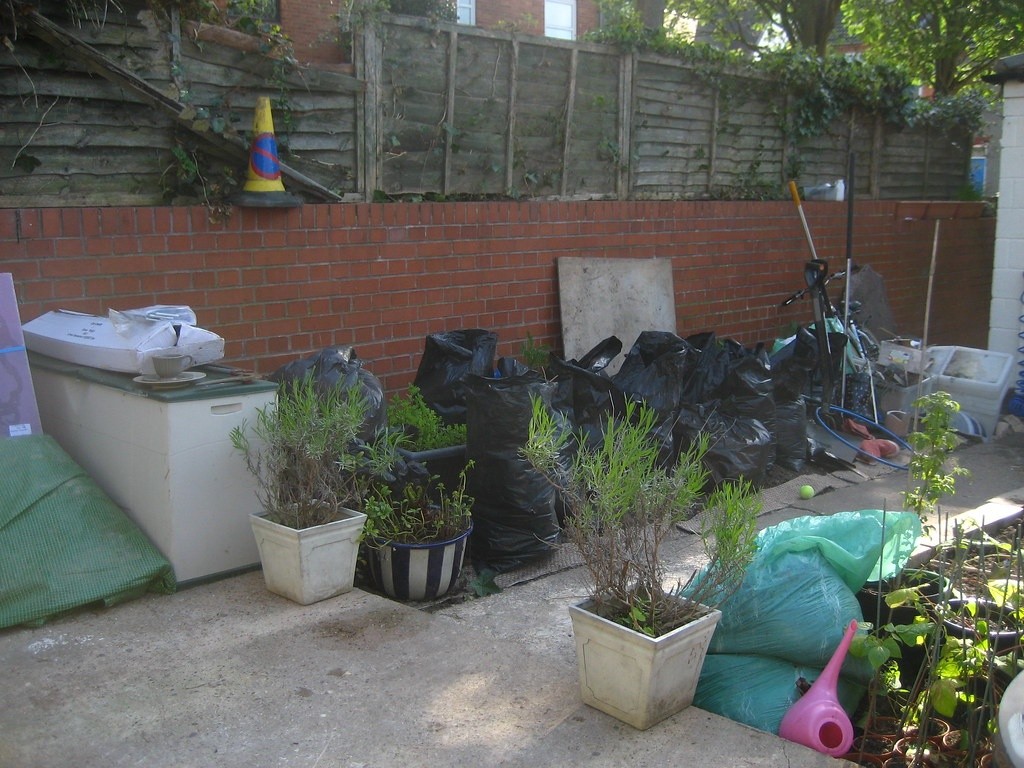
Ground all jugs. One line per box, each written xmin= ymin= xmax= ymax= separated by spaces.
xmin=778 ymin=619 xmax=859 ymax=758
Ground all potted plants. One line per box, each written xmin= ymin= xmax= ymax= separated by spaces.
xmin=385 ymin=382 xmax=467 ymax=505
xmin=521 ymin=393 xmax=765 ymax=732
xmin=842 ymin=519 xmax=1024 ymax=768
xmin=353 ymin=459 xmax=476 ymax=601
xmin=226 ymin=362 xmax=419 ymax=608
xmin=853 ymin=389 xmax=974 ymax=689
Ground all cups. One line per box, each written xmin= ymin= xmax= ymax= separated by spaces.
xmin=152 ymin=354 xmax=192 ymax=378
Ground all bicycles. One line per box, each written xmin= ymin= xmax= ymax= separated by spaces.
xmin=785 ymin=260 xmax=878 ymax=359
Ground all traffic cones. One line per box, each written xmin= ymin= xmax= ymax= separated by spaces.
xmin=233 ymin=96 xmax=304 ymax=208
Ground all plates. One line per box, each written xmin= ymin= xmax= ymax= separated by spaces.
xmin=132 ymin=372 xmax=206 ymax=386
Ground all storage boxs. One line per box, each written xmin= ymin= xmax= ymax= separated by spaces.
xmin=19 ymin=308 xmax=225 ymax=375
xmin=877 ymin=338 xmax=936 ymax=375
xmin=927 ymin=345 xmax=1015 ymax=439
xmin=870 ymin=375 xmax=938 ymax=416
